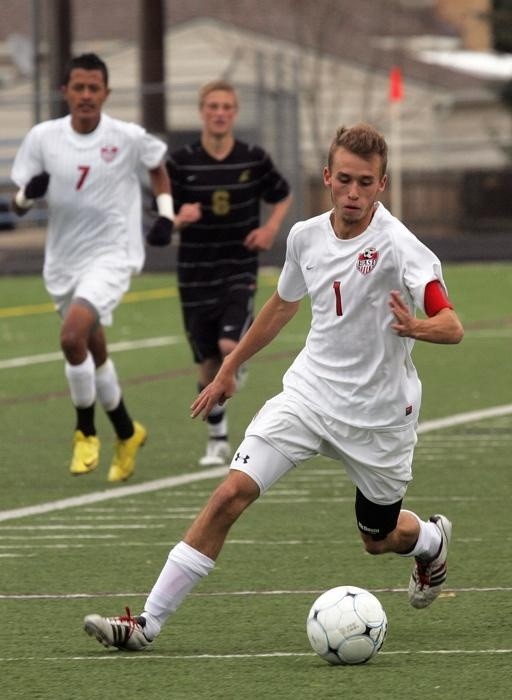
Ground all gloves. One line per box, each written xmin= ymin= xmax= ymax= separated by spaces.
xmin=146 ymin=217 xmax=174 ymax=248
xmin=24 ymin=170 xmax=51 ymax=201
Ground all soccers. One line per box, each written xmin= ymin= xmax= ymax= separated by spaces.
xmin=307 ymin=585 xmax=388 ymax=666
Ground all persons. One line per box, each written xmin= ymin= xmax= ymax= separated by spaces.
xmin=7 ymin=52 xmax=176 ymax=484
xmin=147 ymin=82 xmax=292 ymax=467
xmin=81 ymin=124 xmax=464 ymax=650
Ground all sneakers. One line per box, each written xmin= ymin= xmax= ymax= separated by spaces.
xmin=408 ymin=514 xmax=453 ymax=609
xmin=68 ymin=430 xmax=101 ymax=475
xmin=231 ymin=361 xmax=249 ymax=393
xmin=198 ymin=441 xmax=231 ymax=467
xmin=83 ymin=613 xmax=161 ymax=653
xmin=107 ymin=420 xmax=147 ymax=483
